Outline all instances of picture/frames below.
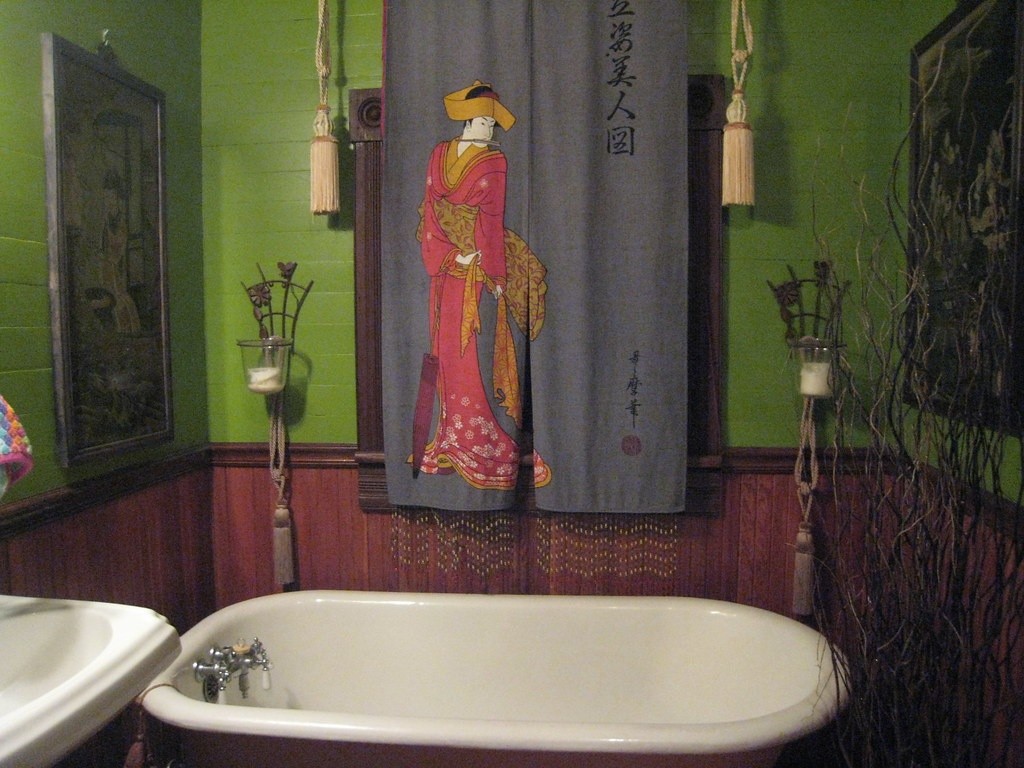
[42,30,175,467]
[904,0,1024,433]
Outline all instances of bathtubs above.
[0,591,182,767]
[141,588,856,768]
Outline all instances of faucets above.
[231,636,273,698]
